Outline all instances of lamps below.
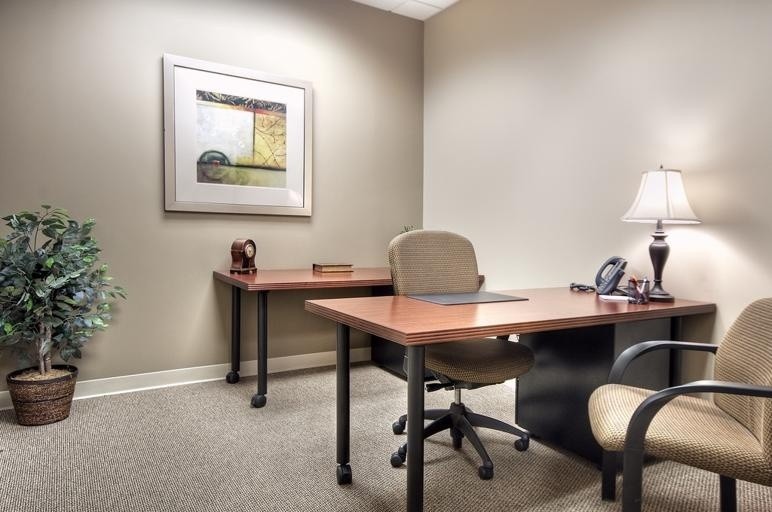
[621,164,702,302]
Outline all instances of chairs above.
[389,228,534,481]
[586,296,771,511]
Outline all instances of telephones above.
[596,256,628,295]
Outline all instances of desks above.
[304,287,716,512]
[214,267,487,408]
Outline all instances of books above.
[312,262,354,274]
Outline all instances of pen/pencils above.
[630,275,648,304]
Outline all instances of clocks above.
[230,239,257,275]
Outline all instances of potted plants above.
[0,202,127,426]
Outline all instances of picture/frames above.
[160,50,315,220]
